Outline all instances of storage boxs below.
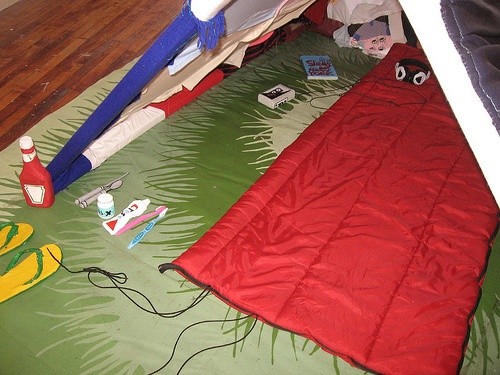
[257,85,296,110]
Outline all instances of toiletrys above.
[18,136,55,208]
[96,192,151,235]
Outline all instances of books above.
[299,54,341,83]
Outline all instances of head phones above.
[394,58,431,86]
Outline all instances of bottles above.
[18,136,55,209]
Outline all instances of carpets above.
[1,30,498,375]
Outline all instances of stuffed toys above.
[350,20,393,61]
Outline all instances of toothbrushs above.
[115,205,169,249]
